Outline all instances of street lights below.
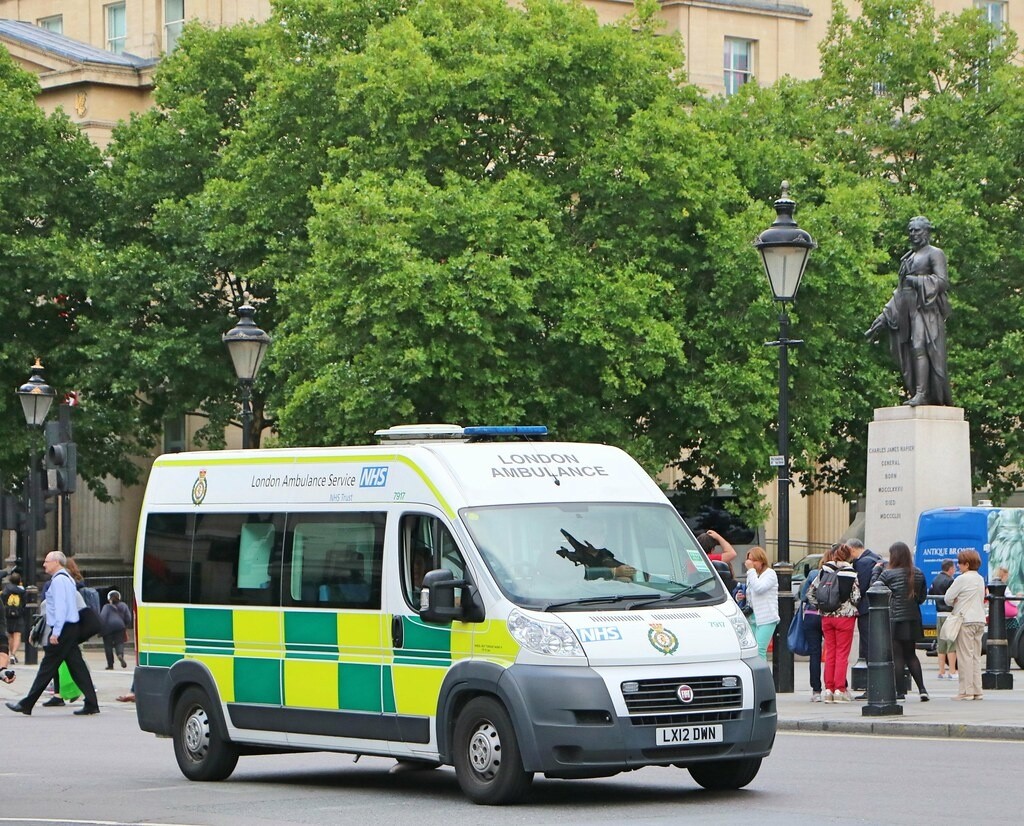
[224,290,270,449]
[752,179,819,692]
[14,357,58,665]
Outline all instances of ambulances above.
[130,424,779,808]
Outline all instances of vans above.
[912,505,1024,670]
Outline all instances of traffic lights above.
[49,443,67,490]
[40,489,56,528]
[15,511,24,534]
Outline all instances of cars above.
[788,553,830,612]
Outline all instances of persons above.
[985,567,1023,672]
[800,538,890,704]
[5,551,100,716]
[0,567,26,664]
[412,550,428,608]
[944,551,987,701]
[928,559,959,680]
[0,597,16,683]
[734,547,780,661]
[685,531,739,592]
[865,216,955,407]
[98,591,132,670]
[870,541,930,702]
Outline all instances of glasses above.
[747,557,758,563]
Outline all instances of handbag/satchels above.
[940,613,964,642]
[124,632,130,643]
[786,606,809,655]
[77,608,104,645]
[27,614,46,648]
[1004,600,1018,617]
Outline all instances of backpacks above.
[815,566,846,613]
[3,587,26,620]
[79,586,100,617]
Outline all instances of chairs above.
[318,583,369,603]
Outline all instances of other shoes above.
[115,694,136,702]
[810,690,868,703]
[6,703,32,715]
[43,682,79,707]
[93,684,98,692]
[950,693,984,701]
[118,655,127,668]
[896,693,905,701]
[938,671,959,679]
[8,654,16,664]
[73,701,100,715]
[105,666,113,670]
[919,689,929,702]
